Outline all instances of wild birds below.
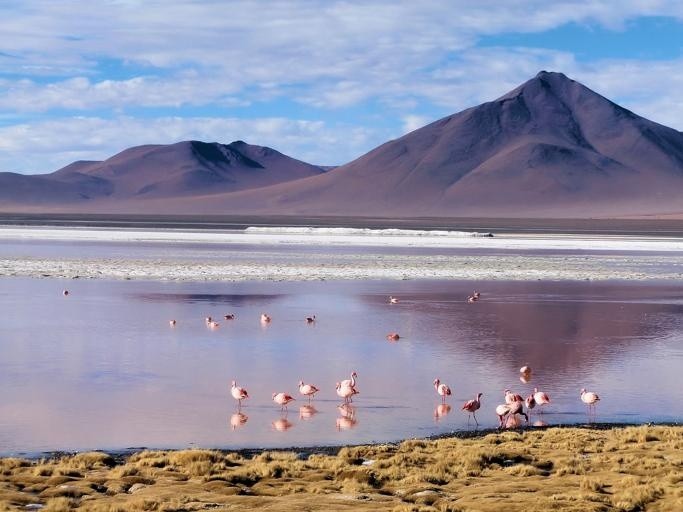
[385,333,399,342]
[467,289,480,303]
[305,315,316,324]
[580,388,601,416]
[299,400,319,422]
[224,315,234,321]
[336,401,357,430]
[229,407,247,434]
[334,372,359,400]
[230,380,248,406]
[387,295,399,306]
[206,317,220,330]
[169,320,176,326]
[272,392,295,412]
[271,411,291,434]
[519,365,531,374]
[519,374,531,384]
[433,378,451,402]
[433,402,451,423]
[297,380,319,400]
[260,314,271,327]
[464,393,483,425]
[497,387,551,429]
